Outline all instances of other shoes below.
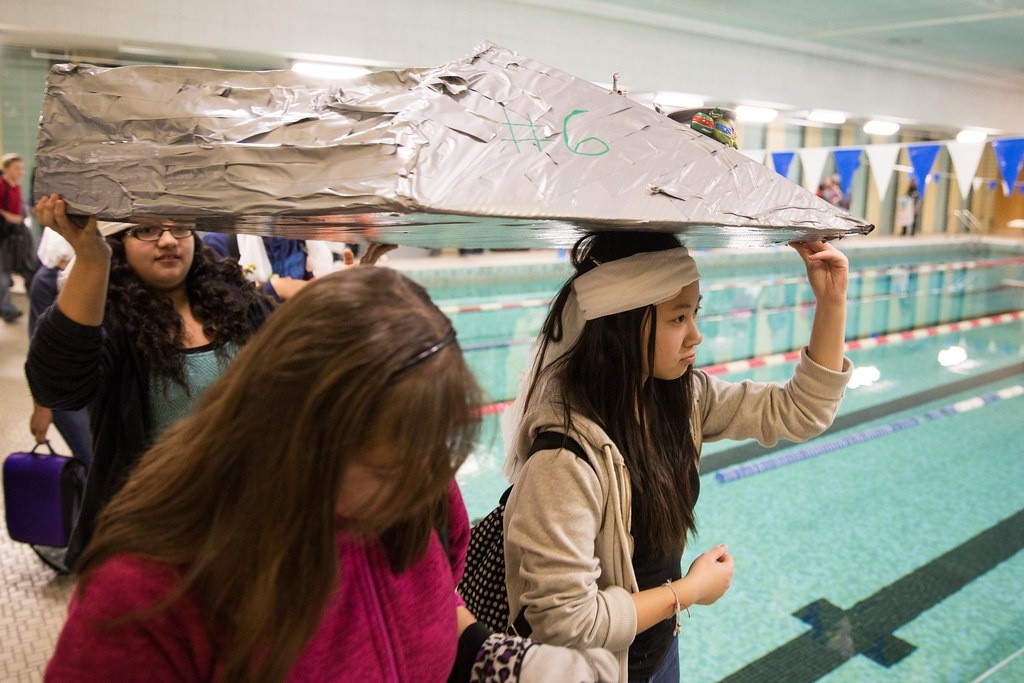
[0,309,24,321]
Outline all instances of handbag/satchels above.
[2,441,88,575]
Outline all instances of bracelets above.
[661,579,692,638]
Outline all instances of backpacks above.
[457,431,598,642]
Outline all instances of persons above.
[502,221,849,683]
[815,173,850,208]
[41,265,596,683]
[26,232,94,472]
[196,228,356,303]
[24,192,399,565]
[0,153,46,321]
[898,179,920,234]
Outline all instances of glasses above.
[123,224,197,242]
